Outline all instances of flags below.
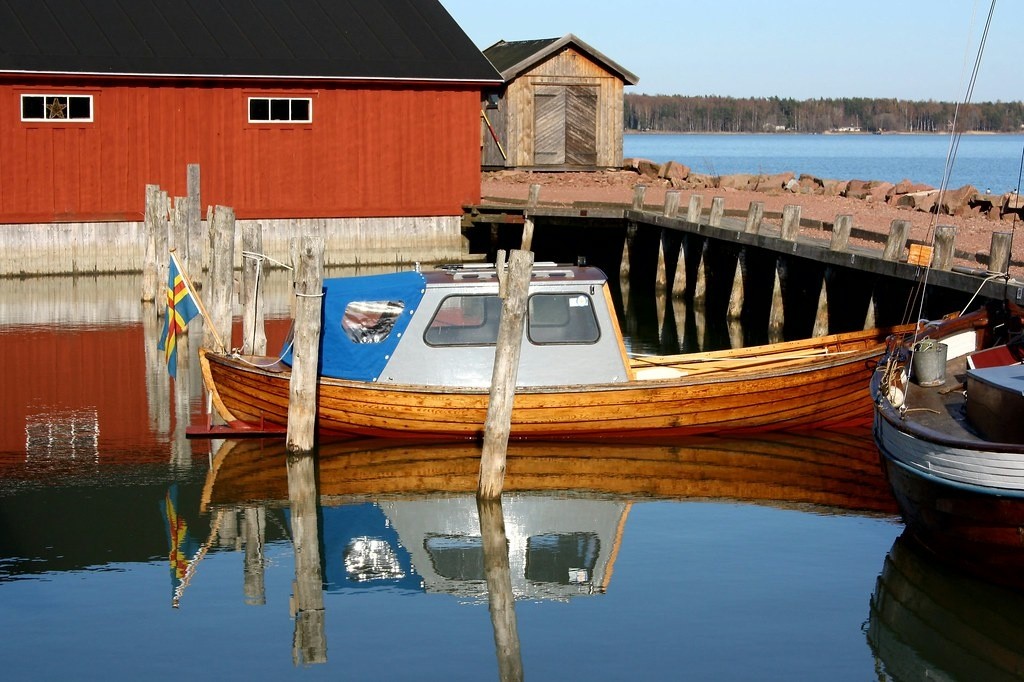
[158,256,200,382]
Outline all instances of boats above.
[870,0,1024,556]
[185,249,990,447]
[198,417,903,521]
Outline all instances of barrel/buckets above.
[908,343,948,387]
